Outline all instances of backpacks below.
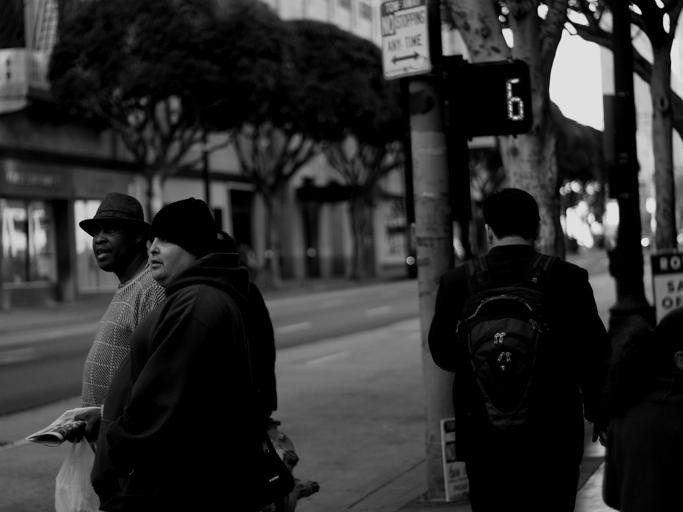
[454,249,562,434]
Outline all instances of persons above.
[425,187,609,512]
[72,198,280,512]
[49,191,164,512]
[602,306,682,512]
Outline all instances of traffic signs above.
[378,0,433,82]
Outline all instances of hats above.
[79,192,153,241]
[149,196,219,260]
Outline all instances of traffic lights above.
[464,57,534,139]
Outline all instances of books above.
[25,405,98,447]
